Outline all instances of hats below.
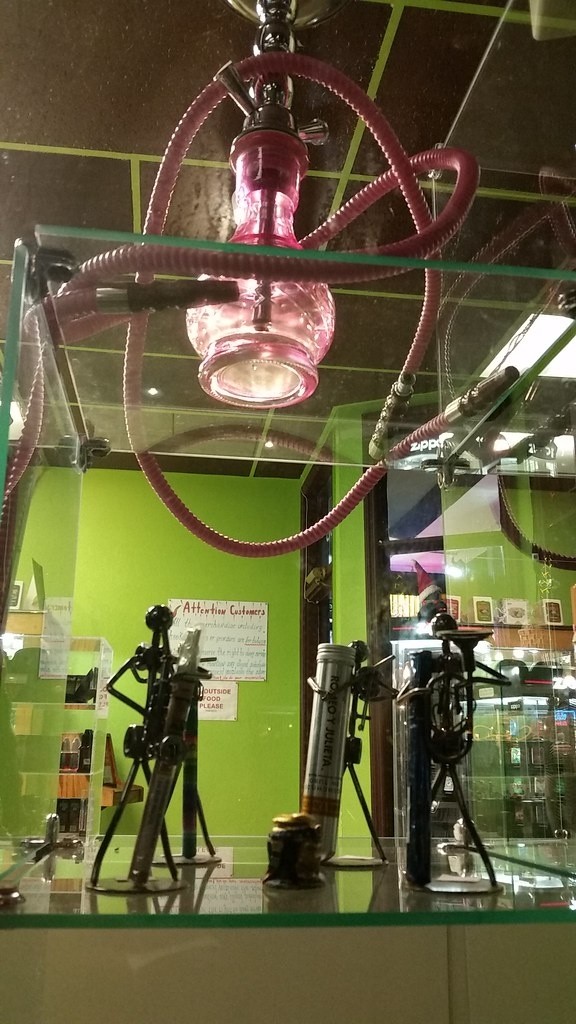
[409,559,441,604]
